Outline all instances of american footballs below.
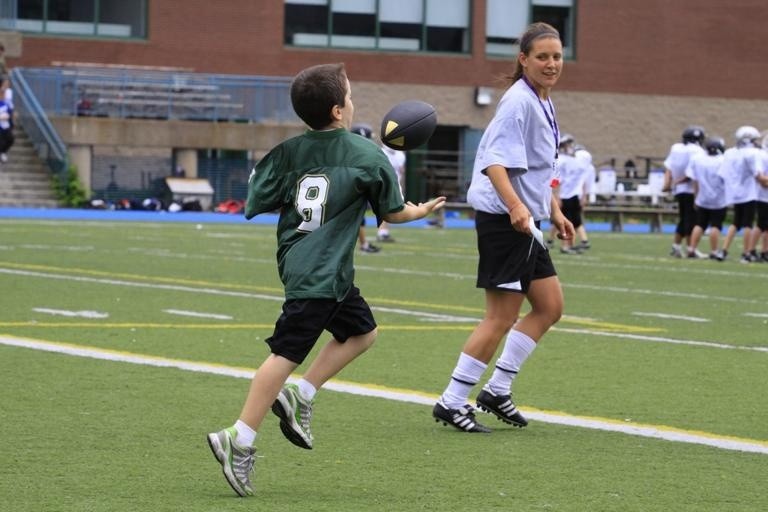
[380,101,436,150]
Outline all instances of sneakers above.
[429,396,491,434]
[267,383,315,451]
[668,240,767,266]
[205,426,258,499]
[475,382,528,428]
[376,235,395,244]
[358,241,382,255]
[559,238,593,257]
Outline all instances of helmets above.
[734,125,763,144]
[681,126,705,140]
[557,128,573,147]
[708,136,724,154]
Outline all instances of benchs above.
[51,52,247,126]
[410,143,735,233]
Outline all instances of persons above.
[207,63,447,497]
[662,126,768,264]
[433,21,577,434]
[0,46,15,162]
[349,125,406,252]
[551,133,596,254]
